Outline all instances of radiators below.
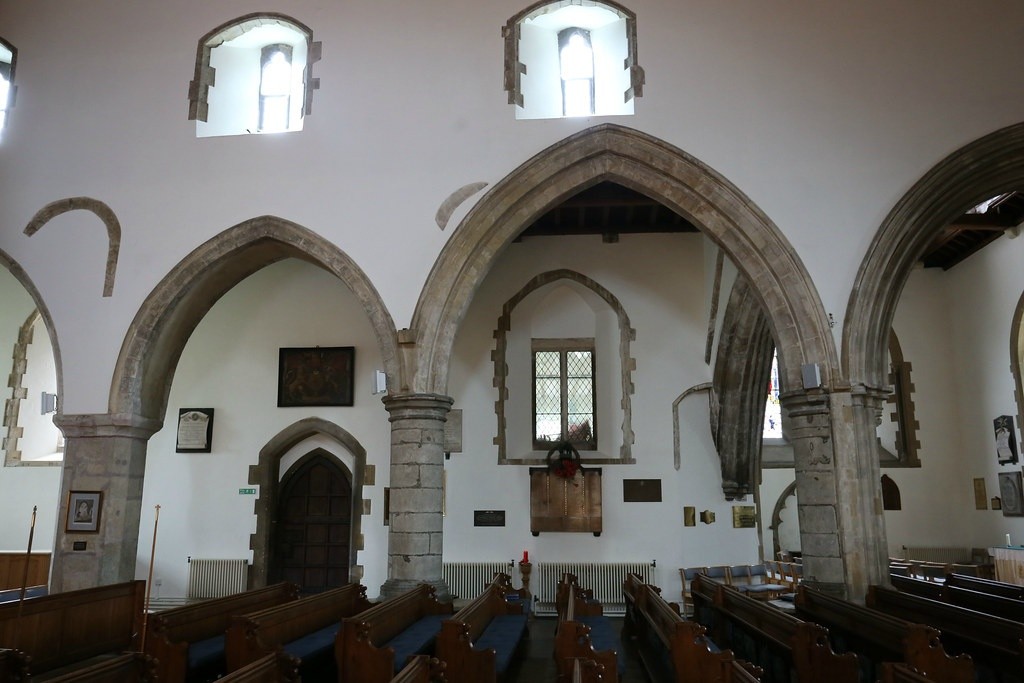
[902,544,972,565]
[537,561,653,603]
[188,556,249,599]
[442,560,512,599]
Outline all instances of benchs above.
[0,568,1024,683]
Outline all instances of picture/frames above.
[276,345,355,408]
[65,489,103,534]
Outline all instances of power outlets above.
[155,576,162,586]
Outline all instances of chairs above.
[678,547,981,621]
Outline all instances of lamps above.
[40,391,56,416]
[801,363,821,390]
[371,370,387,394]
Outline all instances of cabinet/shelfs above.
[529,466,603,538]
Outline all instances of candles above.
[1005,534,1010,545]
[523,550,528,562]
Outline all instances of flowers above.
[553,462,577,481]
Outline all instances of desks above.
[993,545,1024,587]
